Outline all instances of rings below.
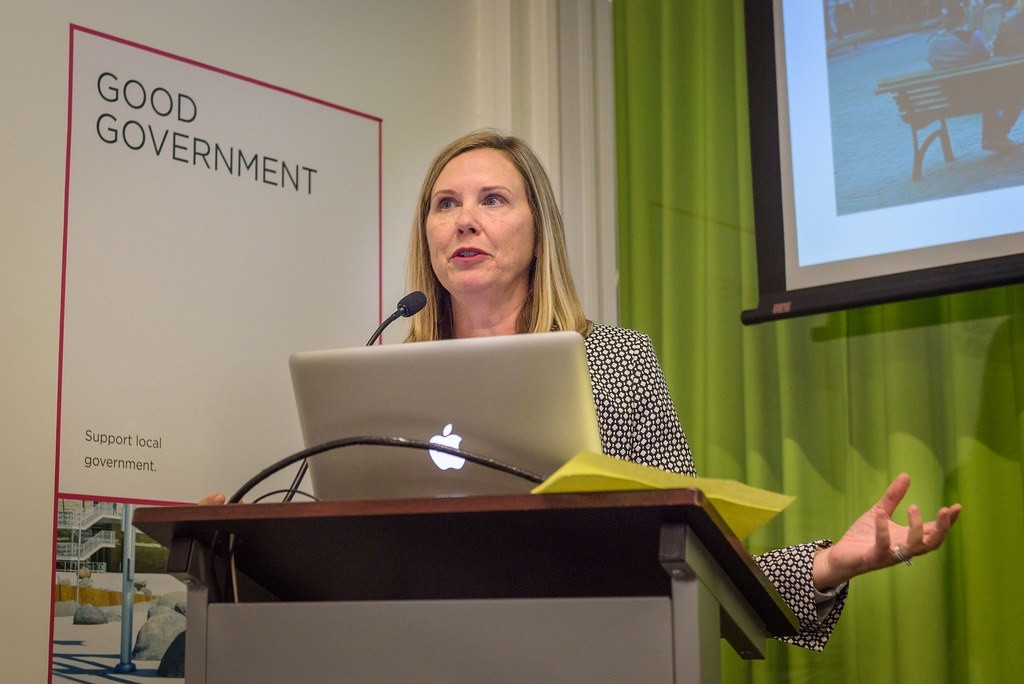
[894,546,912,566]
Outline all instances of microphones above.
[283,290,428,502]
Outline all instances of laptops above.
[289,330,603,504]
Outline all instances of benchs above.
[874,54,1023,179]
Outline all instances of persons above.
[198,128,962,654]
[925,0,1024,151]
